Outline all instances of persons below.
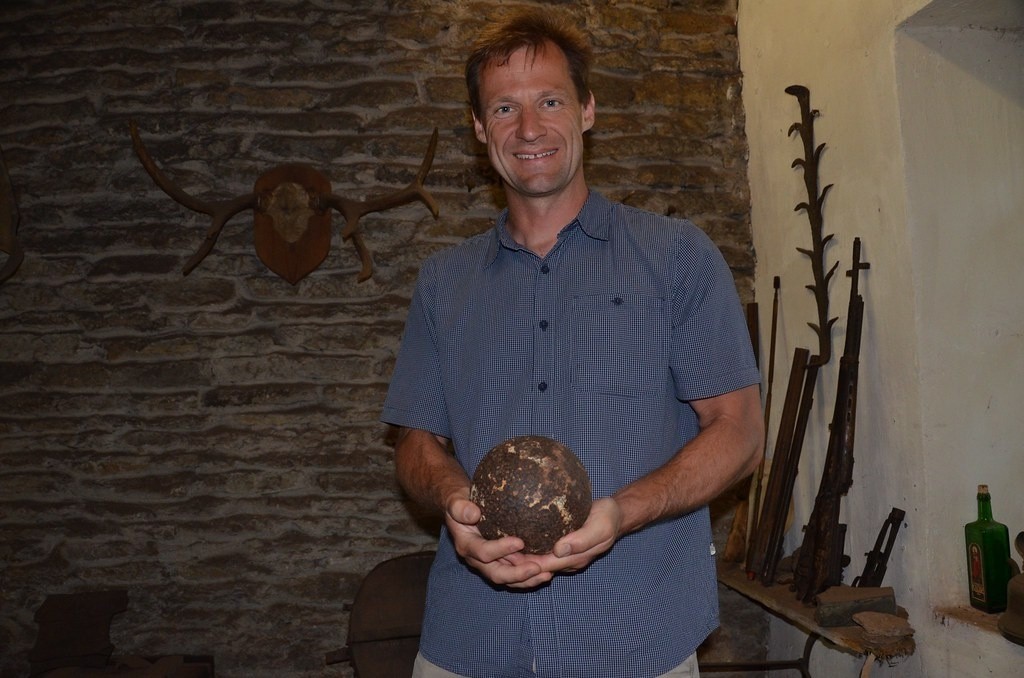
[378,13,766,678]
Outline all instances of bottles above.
[965,492,1011,614]
